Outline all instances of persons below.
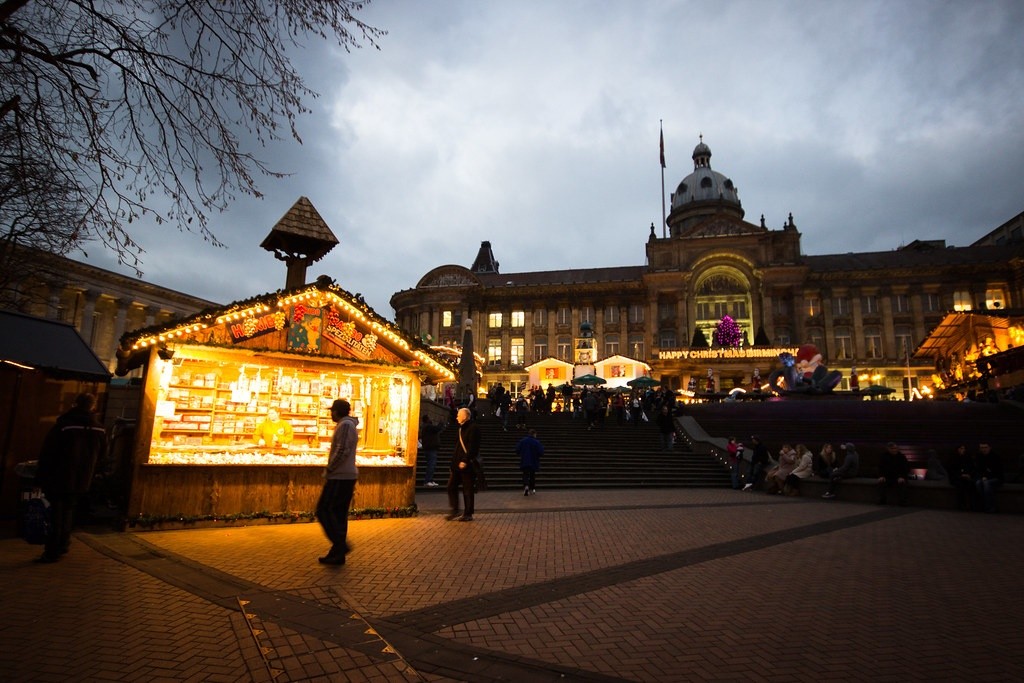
[422,415,444,486]
[656,406,672,449]
[883,441,914,507]
[253,406,294,447]
[767,443,813,496]
[985,383,1024,404]
[948,440,1004,514]
[445,407,481,521]
[812,443,858,501]
[445,382,685,432]
[515,429,544,496]
[316,398,358,565]
[90,419,136,515]
[32,392,108,563]
[726,435,769,491]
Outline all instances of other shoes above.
[455,514,472,523]
[532,489,536,496]
[823,492,835,499]
[446,512,460,520]
[524,486,530,497]
[317,544,352,566]
[33,550,66,562]
[789,489,800,497]
[423,481,439,487]
[743,483,752,491]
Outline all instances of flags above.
[659,131,666,168]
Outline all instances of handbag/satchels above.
[625,410,631,421]
[470,458,483,472]
[496,406,503,418]
[672,433,677,445]
[642,413,648,422]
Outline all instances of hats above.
[328,400,351,416]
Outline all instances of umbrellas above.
[861,385,896,400]
[626,376,664,391]
[571,374,607,388]
